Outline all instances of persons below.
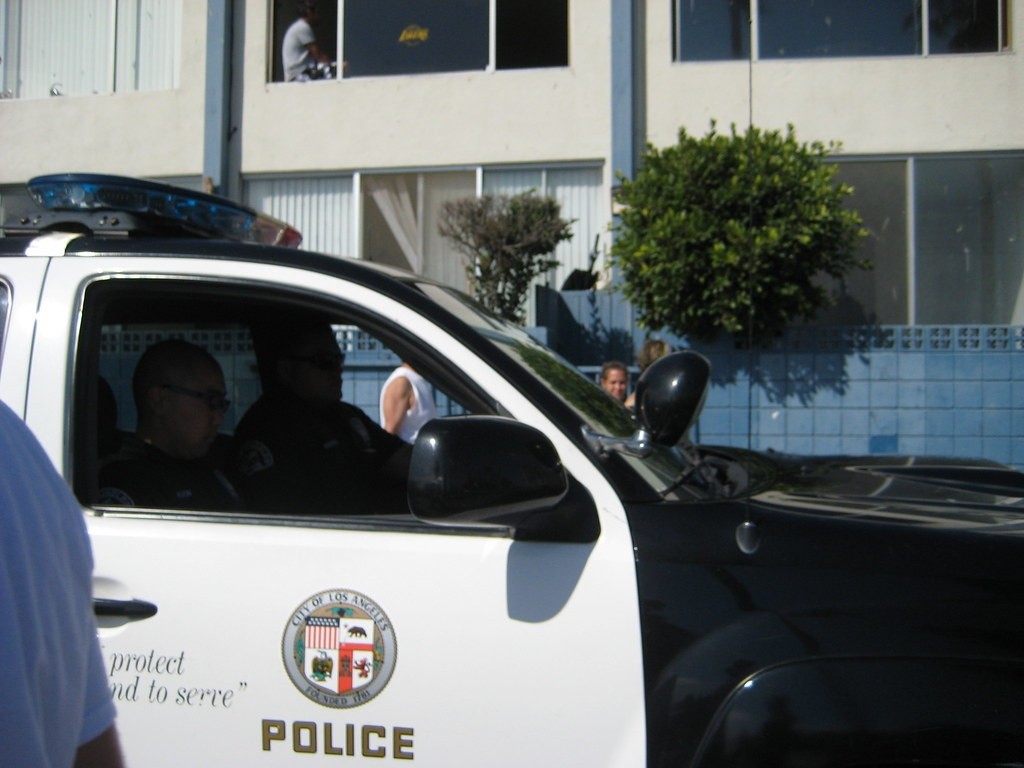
[234,308,413,515]
[281,0,350,82]
[106,339,234,513]
[599,363,627,401]
[625,340,670,408]
[379,361,439,445]
[0,401,127,768]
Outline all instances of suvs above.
[0,172,1023,768]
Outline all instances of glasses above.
[281,352,346,370]
[162,381,230,415]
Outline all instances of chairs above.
[74,366,137,508]
